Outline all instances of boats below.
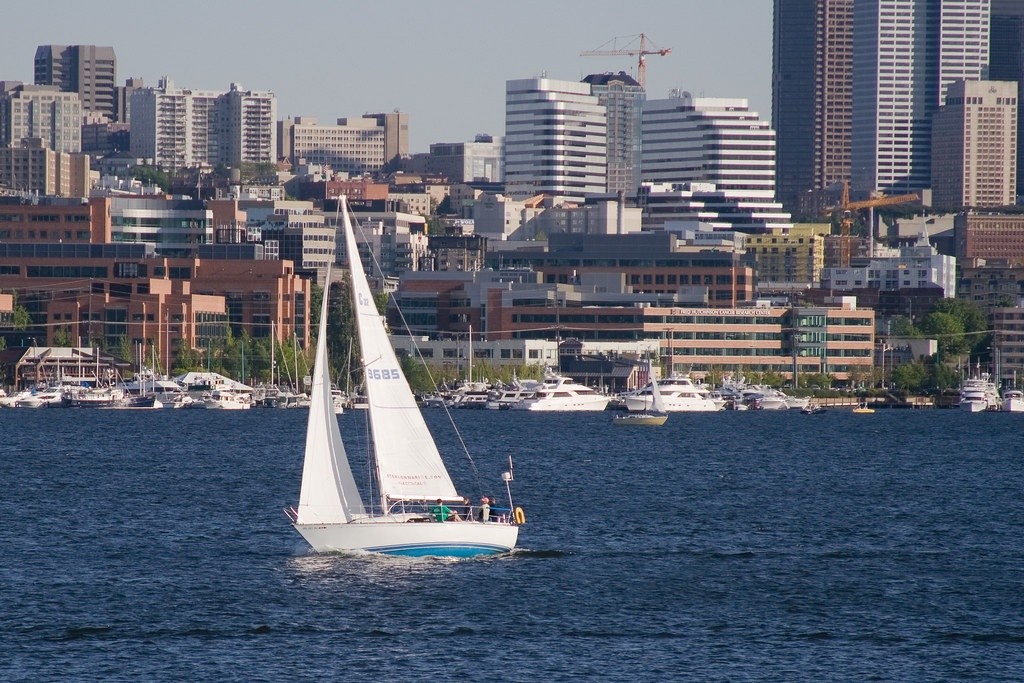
[852,401,876,415]
[1001,388,1024,413]
[957,376,1001,414]
[421,308,828,415]
[611,412,669,427]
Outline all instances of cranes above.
[577,32,673,88]
[820,177,922,269]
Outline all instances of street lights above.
[958,350,971,389]
[882,346,894,388]
[986,346,999,388]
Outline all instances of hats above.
[481,497,489,503]
[489,496,495,505]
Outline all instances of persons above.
[464,498,473,521]
[489,497,504,522]
[479,498,490,521]
[432,499,457,522]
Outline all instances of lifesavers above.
[515,507,525,524]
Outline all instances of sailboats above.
[281,194,523,560]
[0,316,370,416]
